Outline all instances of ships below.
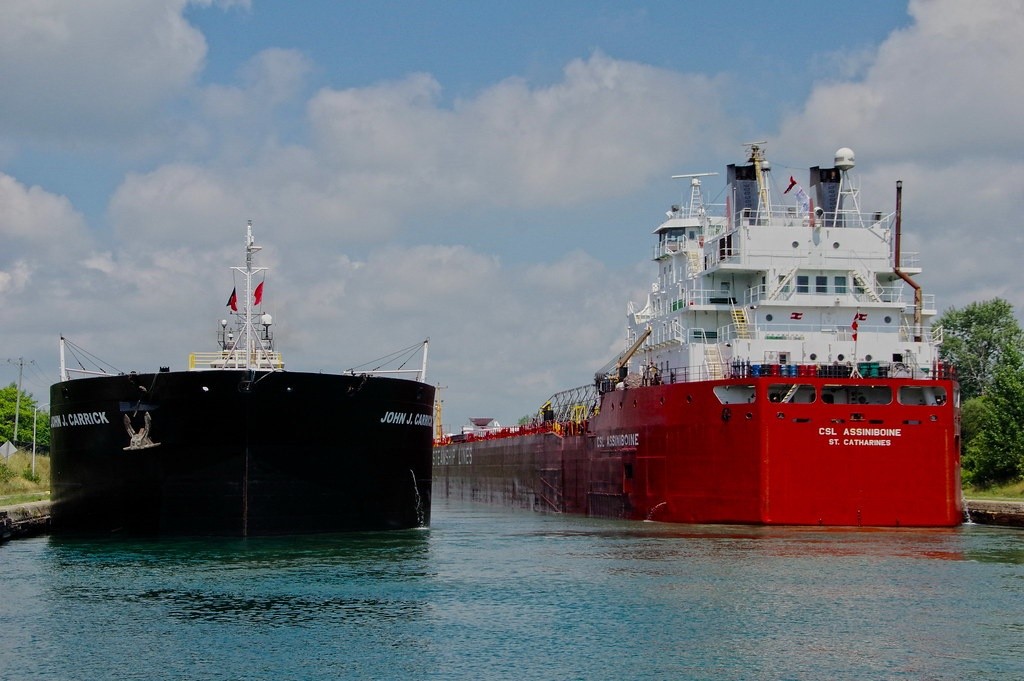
[50,217,436,547]
[433,135,966,526]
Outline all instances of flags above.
[783,176,797,195]
[253,281,263,306]
[853,313,858,321]
[852,333,857,342]
[794,188,807,204]
[851,321,857,331]
[858,314,867,321]
[226,289,237,312]
[790,313,802,320]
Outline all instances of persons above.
[564,425,584,437]
[653,372,675,386]
[225,338,237,351]
